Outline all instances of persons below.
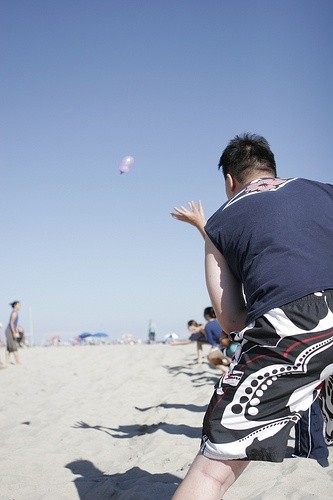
[4,301,22,368]
[204,306,226,352]
[187,319,209,361]
[13,326,24,347]
[207,330,241,375]
[171,133,333,500]
[148,319,155,343]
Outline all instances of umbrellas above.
[93,332,108,336]
[80,333,92,338]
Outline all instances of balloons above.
[119,156,134,174]
